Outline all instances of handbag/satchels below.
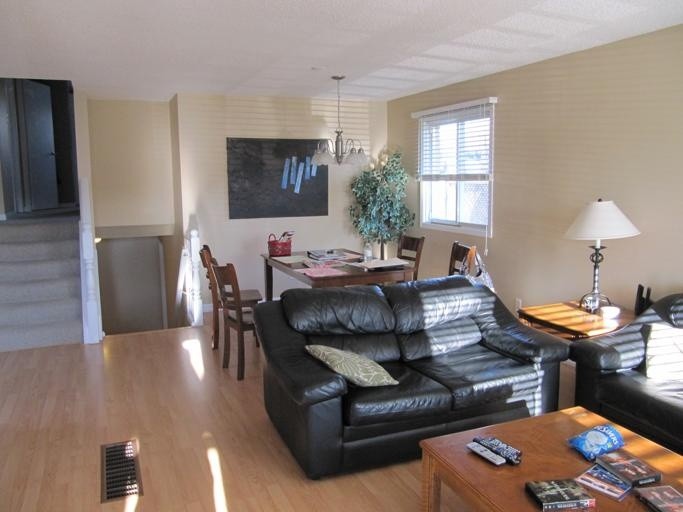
[459,249,494,293]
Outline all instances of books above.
[306,248,348,263]
[523,448,682,512]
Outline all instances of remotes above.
[473,435,520,464]
[486,436,522,456]
[467,442,506,465]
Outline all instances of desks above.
[260,248,416,302]
[517,300,635,341]
[419,406,683,512]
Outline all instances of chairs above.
[211,258,259,380]
[448,240,477,275]
[397,232,425,283]
[199,244,263,349]
[569,293,683,455]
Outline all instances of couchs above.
[252,275,570,480]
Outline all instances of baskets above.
[268,232,291,257]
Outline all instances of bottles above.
[363,242,373,271]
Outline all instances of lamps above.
[561,199,641,313]
[310,75,368,166]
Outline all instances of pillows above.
[280,274,483,387]
[646,322,683,380]
